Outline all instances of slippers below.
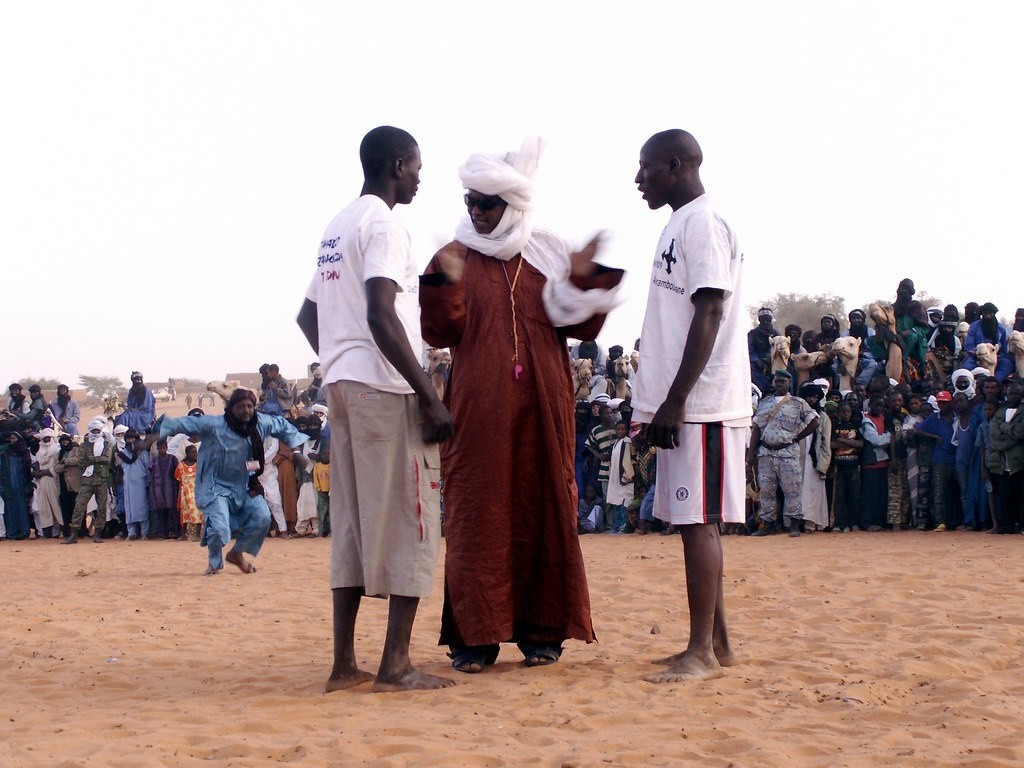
[205,562,223,576]
[225,552,256,573]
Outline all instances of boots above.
[93,529,103,543]
[61,528,78,543]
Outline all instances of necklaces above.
[502,255,523,380]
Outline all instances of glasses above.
[464,194,502,211]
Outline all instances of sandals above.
[526,646,560,666]
[446,647,486,672]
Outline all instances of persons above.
[418,134,626,674]
[630,129,754,683]
[296,125,457,693]
[0,278,1024,576]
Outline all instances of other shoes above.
[607,520,1018,537]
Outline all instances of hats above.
[1002,373,1021,383]
[776,369,792,378]
[936,391,951,402]
[630,420,641,426]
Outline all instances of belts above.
[760,441,794,451]
